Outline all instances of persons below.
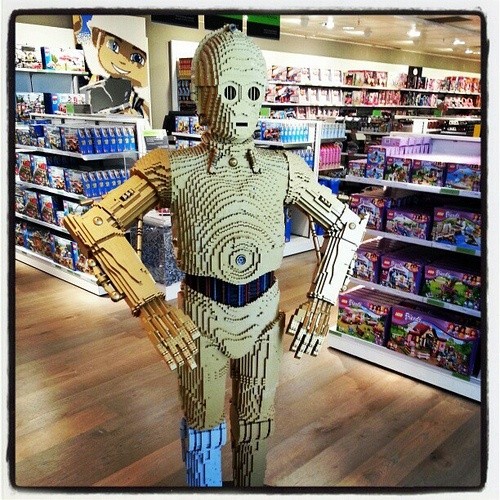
[61,24,369,486]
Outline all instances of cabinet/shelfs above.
[166,110,348,259]
[327,130,481,403]
[169,41,481,156]
[16,68,153,296]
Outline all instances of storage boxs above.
[16,44,481,381]
